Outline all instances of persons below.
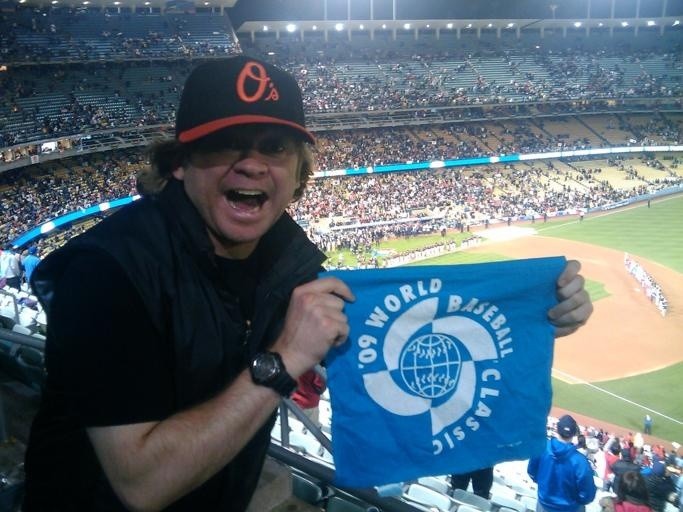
[448,399,683,511]
[17,50,593,511]
[285,354,325,433]
[1,0,683,292]
[647,289,671,319]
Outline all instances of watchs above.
[245,336,297,399]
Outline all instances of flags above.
[315,261,563,485]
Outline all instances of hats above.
[557,416,577,437]
[176,56,316,143]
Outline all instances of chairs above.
[269,415,537,511]
[0,283,47,399]
